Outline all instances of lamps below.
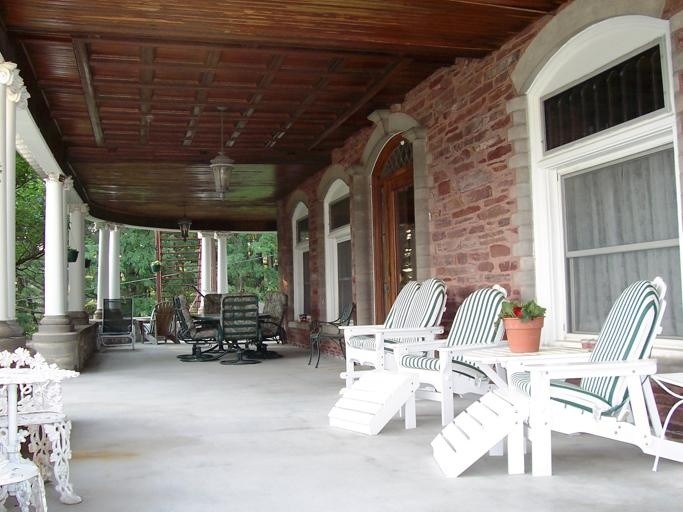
[178,204,192,242]
[209,106,235,201]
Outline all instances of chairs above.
[0,347,81,512]
[308,303,355,368]
[96,291,288,365]
[429,277,672,478]
[329,277,516,439]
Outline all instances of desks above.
[462,344,596,454]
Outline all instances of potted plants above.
[497,295,550,356]
